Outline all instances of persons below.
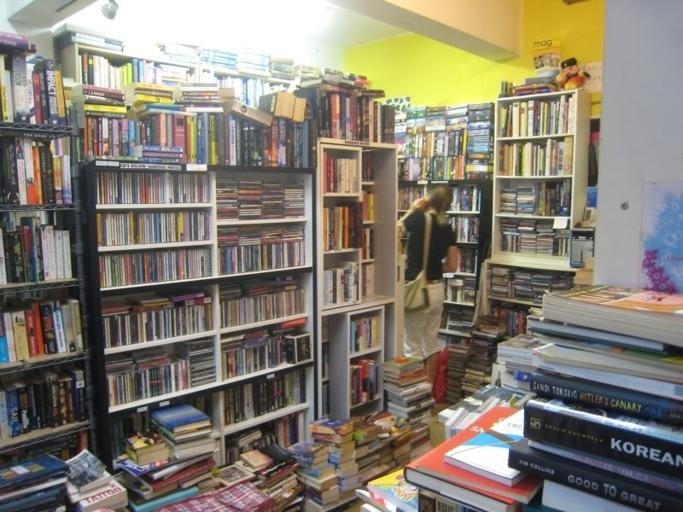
[395,187,458,361]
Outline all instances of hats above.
[560,57,578,68]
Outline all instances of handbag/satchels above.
[403,209,431,314]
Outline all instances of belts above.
[426,279,450,284]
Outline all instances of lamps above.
[102,0,119,19]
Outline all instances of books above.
[322,259,359,309]
[316,85,395,146]
[348,316,383,353]
[217,176,306,220]
[486,266,573,305]
[218,327,313,379]
[572,232,595,270]
[112,395,216,460]
[322,317,334,422]
[78,52,314,167]
[154,32,370,90]
[362,226,375,260]
[0,365,87,441]
[99,247,211,289]
[104,337,218,409]
[397,181,479,213]
[499,69,557,99]
[218,224,308,274]
[364,263,376,301]
[212,357,435,512]
[95,170,210,204]
[220,274,308,328]
[323,199,361,252]
[322,148,359,193]
[497,178,572,217]
[112,404,215,512]
[94,210,211,246]
[399,212,480,243]
[495,216,569,256]
[395,102,494,180]
[0,210,77,285]
[220,366,311,427]
[362,149,377,183]
[363,189,376,224]
[347,284,682,512]
[1,291,83,365]
[0,448,129,512]
[0,29,78,131]
[1,132,75,208]
[498,94,573,139]
[224,410,308,468]
[454,245,477,275]
[100,284,214,350]
[0,430,90,467]
[497,137,573,177]
[445,276,475,303]
[51,24,125,52]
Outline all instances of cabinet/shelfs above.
[491,88,591,263]
[310,135,401,420]
[483,257,594,340]
[398,179,493,345]
[81,159,315,476]
[1,121,103,467]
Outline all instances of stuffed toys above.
[557,57,590,92]
